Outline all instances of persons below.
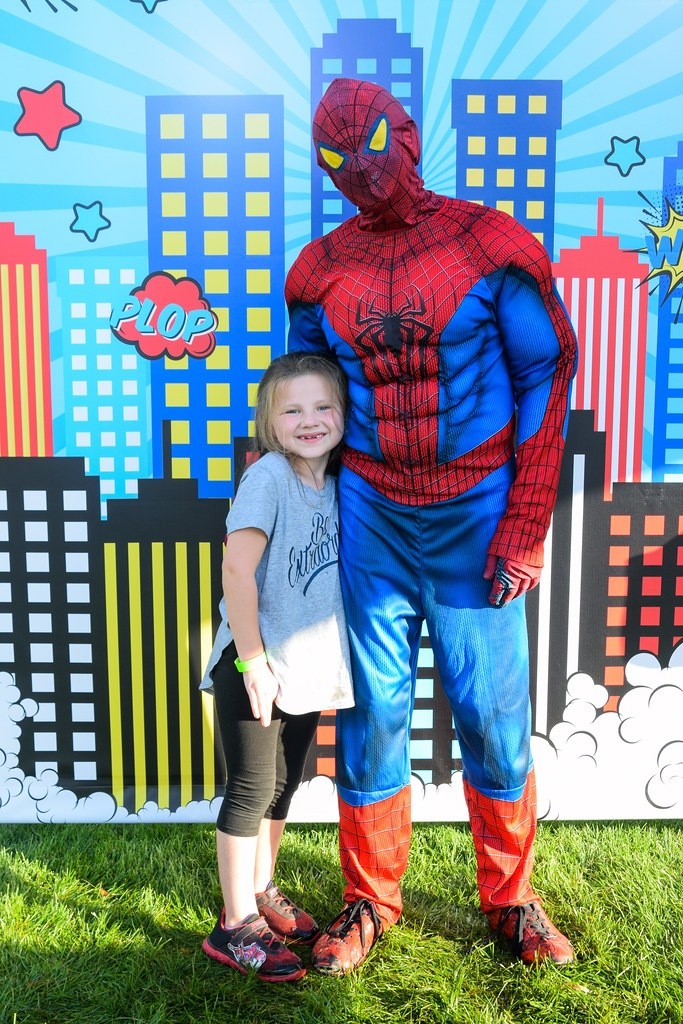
[199,356,356,983]
[286,76,577,973]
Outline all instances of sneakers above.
[310,898,382,976]
[254,881,319,946]
[201,904,306,982]
[488,899,574,965]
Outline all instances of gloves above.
[483,557,537,607]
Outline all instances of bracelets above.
[234,651,267,672]
